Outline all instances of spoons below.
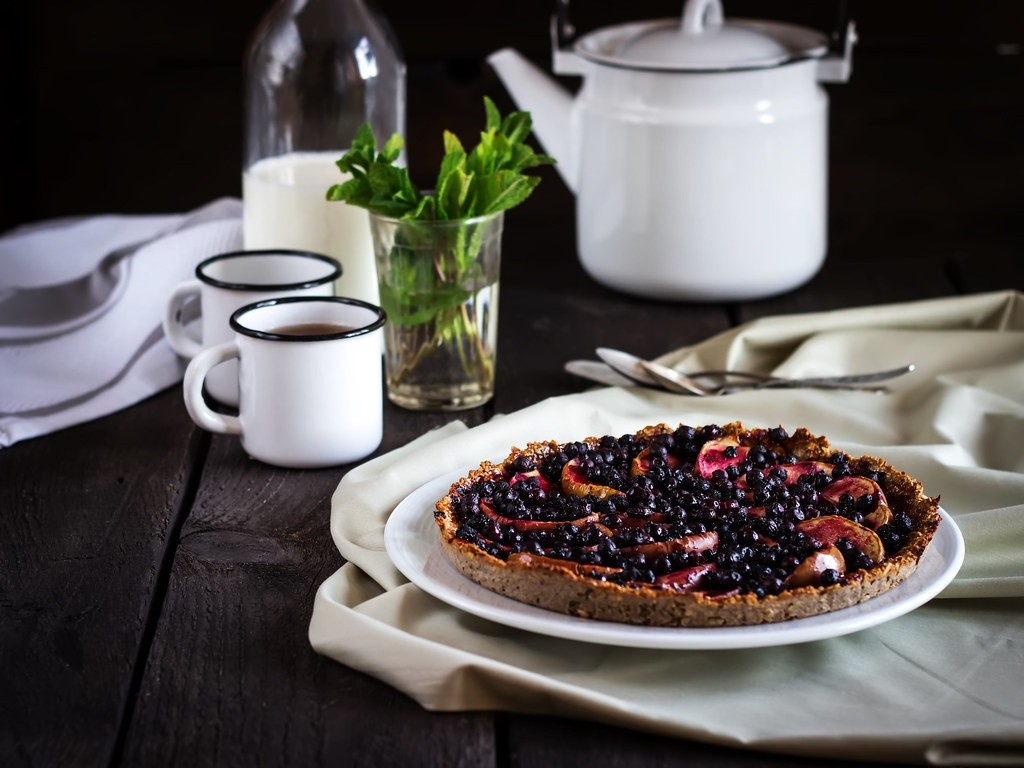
[564,346,916,396]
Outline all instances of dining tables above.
[0,198,820,768]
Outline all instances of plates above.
[384,460,966,649]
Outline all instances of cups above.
[163,249,342,404]
[370,190,504,411]
[183,296,384,467]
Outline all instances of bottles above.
[241,0,405,309]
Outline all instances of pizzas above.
[435,425,942,627]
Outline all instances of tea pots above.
[486,0,859,301]
